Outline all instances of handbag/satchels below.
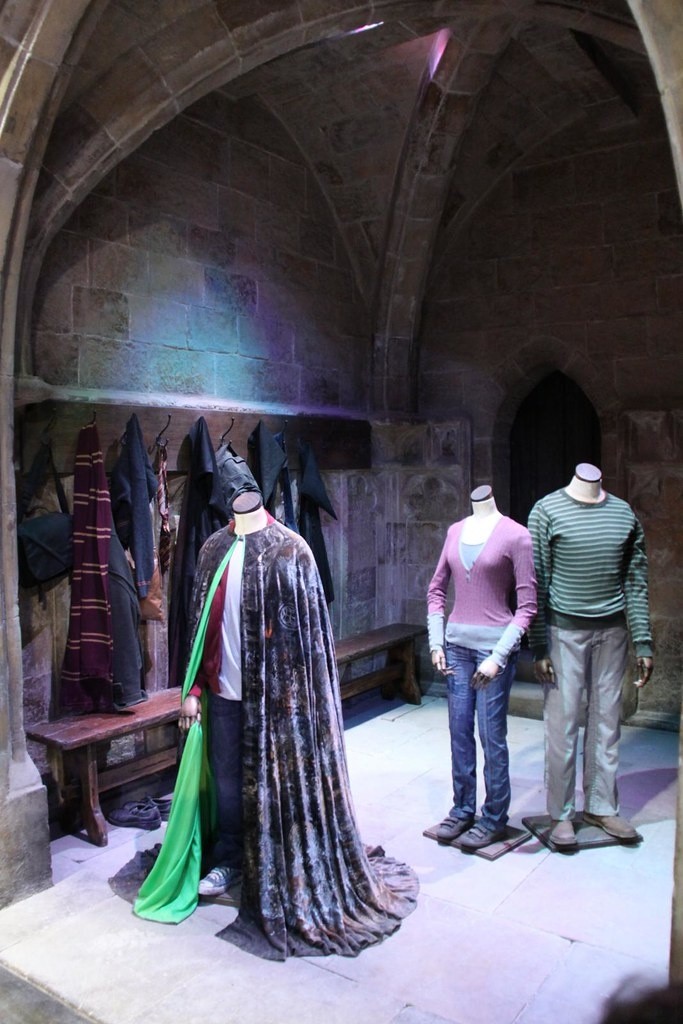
[18,511,74,590]
[121,548,164,624]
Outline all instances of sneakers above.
[199,864,242,895]
[436,816,474,839]
[460,823,507,849]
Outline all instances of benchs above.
[335,623,427,705]
[25,686,183,847]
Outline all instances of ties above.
[157,443,171,588]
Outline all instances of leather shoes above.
[582,810,638,840]
[107,795,172,830]
[548,820,578,847]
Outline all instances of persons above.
[528,464,655,845]
[116,491,420,961]
[426,484,538,847]
